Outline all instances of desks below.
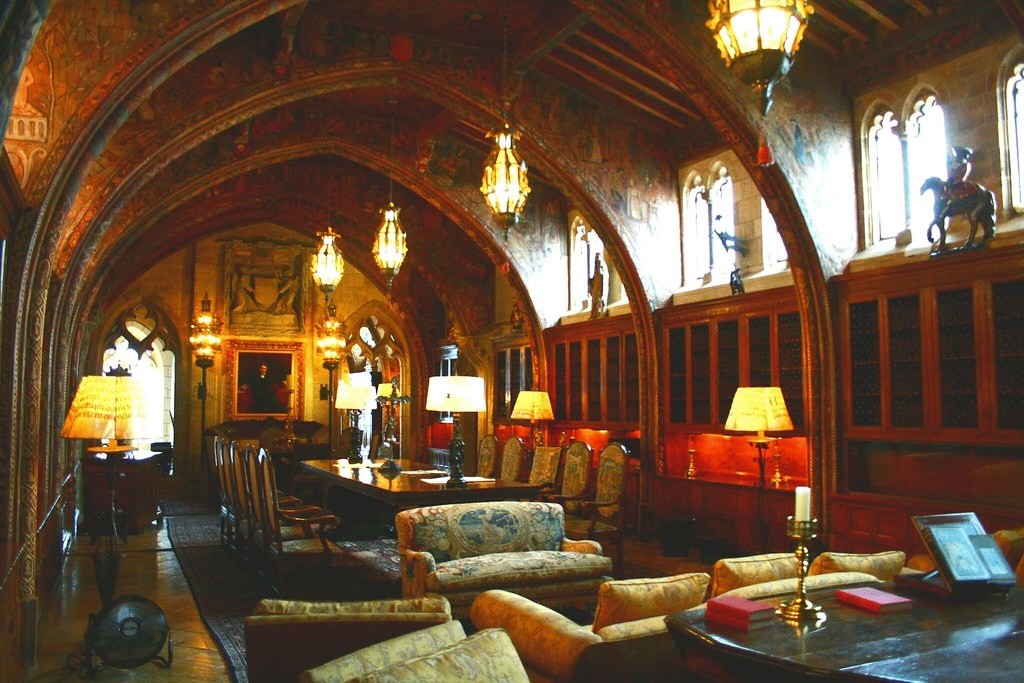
[300,455,544,513]
[664,569,1021,682]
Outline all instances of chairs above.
[476,435,631,579]
[339,428,364,459]
[204,430,355,600]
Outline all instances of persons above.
[274,265,295,314]
[231,265,255,312]
[940,147,972,218]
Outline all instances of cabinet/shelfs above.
[653,286,806,554]
[81,449,164,535]
[491,333,533,480]
[543,313,642,531]
[826,243,1024,567]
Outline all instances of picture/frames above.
[222,238,309,338]
[224,339,306,422]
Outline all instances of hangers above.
[159,500,236,515]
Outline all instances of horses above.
[920,176,998,250]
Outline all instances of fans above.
[85,595,173,681]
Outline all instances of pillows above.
[593,573,711,633]
[713,550,797,599]
[806,550,905,581]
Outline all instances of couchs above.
[240,501,1024,683]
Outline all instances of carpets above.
[167,516,718,683]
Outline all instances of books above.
[704,595,775,631]
[834,587,912,613]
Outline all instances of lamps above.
[59,375,163,606]
[480,16,530,276]
[509,391,554,448]
[308,157,344,323]
[335,378,369,463]
[183,290,223,483]
[426,376,486,489]
[723,386,795,554]
[315,299,348,459]
[706,1,814,167]
[372,98,410,310]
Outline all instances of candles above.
[794,487,812,521]
